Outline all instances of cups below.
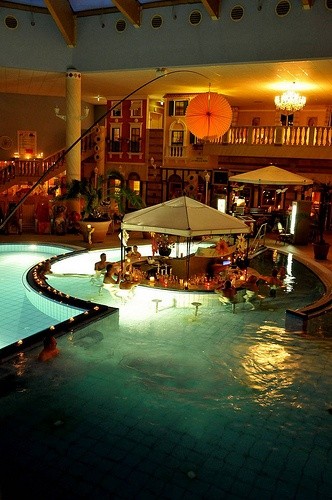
[150,277,154,286]
[179,278,187,289]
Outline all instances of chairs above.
[275,222,293,246]
[34,200,54,234]
[4,200,23,234]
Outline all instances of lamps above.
[273,81,308,114]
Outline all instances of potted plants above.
[53,168,149,243]
[308,180,332,259]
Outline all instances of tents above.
[119,196,251,286]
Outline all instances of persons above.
[110,274,139,302]
[38,336,61,363]
[98,264,123,294]
[240,275,259,311]
[38,260,52,280]
[86,227,95,251]
[125,244,141,262]
[95,253,118,271]
[259,265,289,297]
[214,281,237,305]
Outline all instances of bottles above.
[156,265,178,287]
